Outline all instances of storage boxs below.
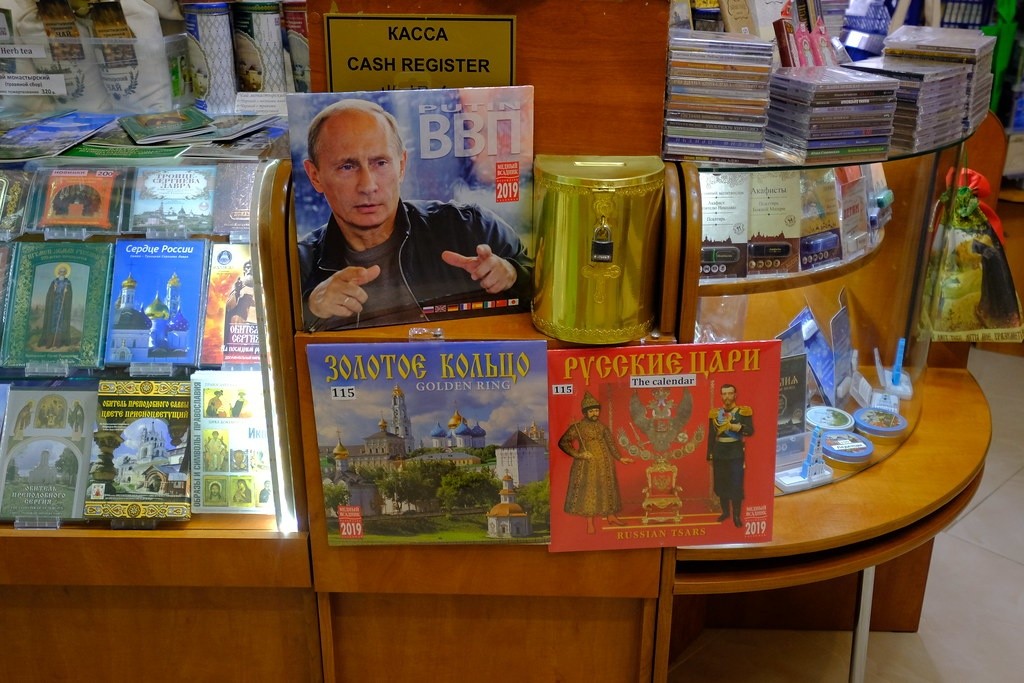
[535,153,666,346]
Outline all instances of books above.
[0,107,289,523]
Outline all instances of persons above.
[297,99,534,332]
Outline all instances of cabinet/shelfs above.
[973,187,1024,358]
[0,159,325,683]
[651,127,994,683]
[288,153,683,683]
